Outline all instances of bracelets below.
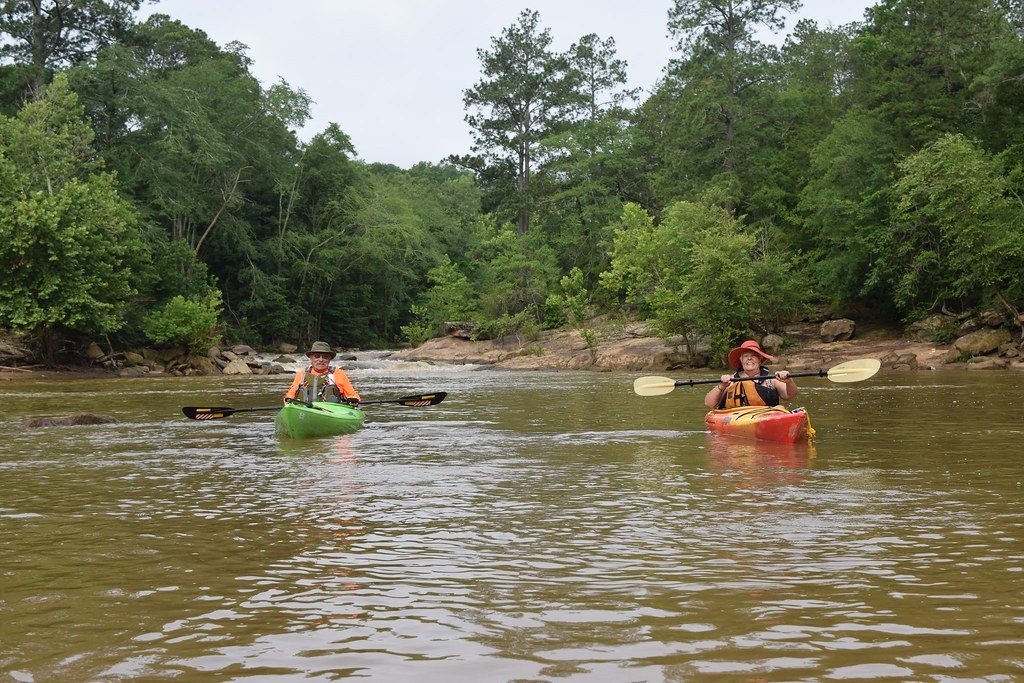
[718,385,724,391]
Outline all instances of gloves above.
[347,398,358,407]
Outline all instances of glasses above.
[314,354,328,359]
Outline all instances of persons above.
[705,340,798,411]
[283,341,361,408]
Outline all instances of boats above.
[703,406,807,444]
[274,399,365,438]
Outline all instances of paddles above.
[181,387,448,424]
[633,357,884,398]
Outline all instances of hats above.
[728,340,773,370]
[306,341,336,360]
